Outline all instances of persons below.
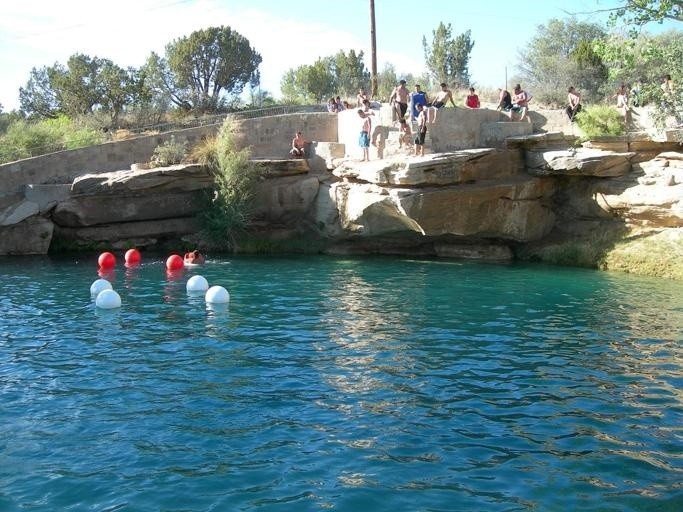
[564,86,582,125]
[184,249,205,264]
[289,132,313,159]
[327,80,532,163]
[611,72,675,122]
